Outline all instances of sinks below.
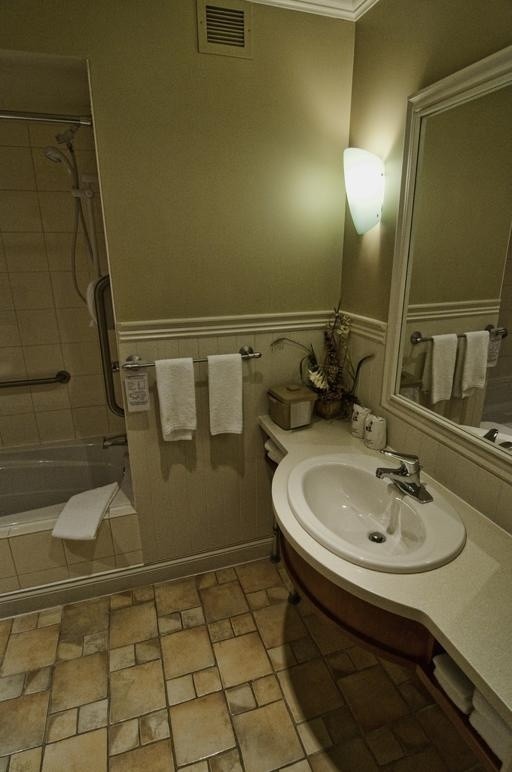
[1,446,133,536]
[462,424,511,451]
[288,454,466,573]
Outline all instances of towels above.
[50,482,120,542]
[154,356,198,444]
[421,329,491,406]
[431,654,508,763]
[206,351,244,437]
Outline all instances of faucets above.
[102,434,126,451]
[376,449,433,504]
[484,428,512,448]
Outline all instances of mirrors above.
[379,43,512,486]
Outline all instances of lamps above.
[341,146,384,236]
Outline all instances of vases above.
[315,399,343,420]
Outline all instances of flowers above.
[269,298,375,401]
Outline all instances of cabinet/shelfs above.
[275,525,511,772]
